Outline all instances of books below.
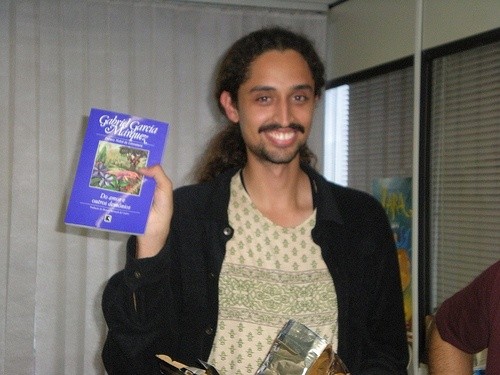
[61,104,170,239]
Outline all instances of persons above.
[423,254,500,375]
[95,24,412,375]
